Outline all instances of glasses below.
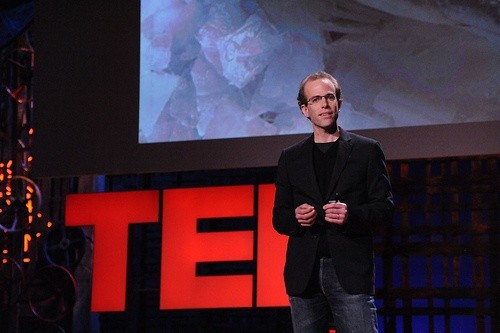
[307,94,336,105]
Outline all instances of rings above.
[336,214,339,219]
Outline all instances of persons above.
[272,72,395,333]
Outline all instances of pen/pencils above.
[335,193,339,202]
